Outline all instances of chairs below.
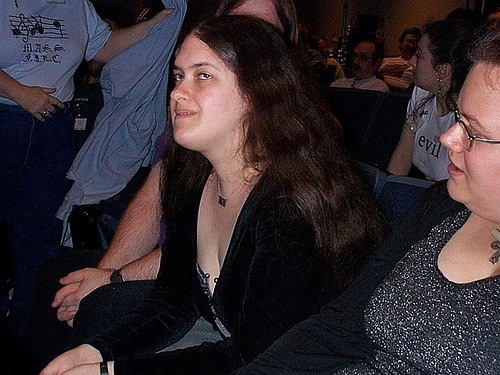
[321,84,437,310]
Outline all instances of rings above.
[42,109,47,116]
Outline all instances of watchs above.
[109,268,125,285]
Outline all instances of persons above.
[374,25,425,89]
[35,12,393,375]
[57,16,119,193]
[309,31,345,86]
[346,10,386,77]
[385,19,482,189]
[330,36,390,93]
[0,0,173,331]
[232,16,500,375]
[19,0,308,375]
[440,7,485,90]
[476,3,500,82]
[292,21,326,88]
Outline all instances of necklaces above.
[213,159,263,209]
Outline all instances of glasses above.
[452,100,500,148]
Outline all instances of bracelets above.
[100,360,110,375]
